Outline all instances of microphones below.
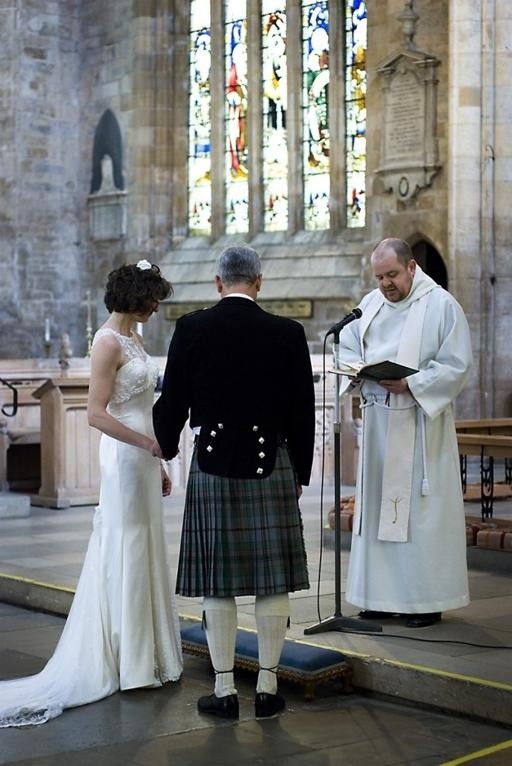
[330,307,362,333]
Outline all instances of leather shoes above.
[358,611,442,628]
[255,693,285,720]
[197,693,239,718]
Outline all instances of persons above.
[331,238,475,627]
[150,247,316,721]
[1,260,185,728]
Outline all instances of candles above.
[86,290,92,325]
[137,322,143,337]
[45,318,50,341]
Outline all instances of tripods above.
[304,332,383,635]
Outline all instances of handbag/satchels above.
[197,414,277,479]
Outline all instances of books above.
[327,358,418,386]
